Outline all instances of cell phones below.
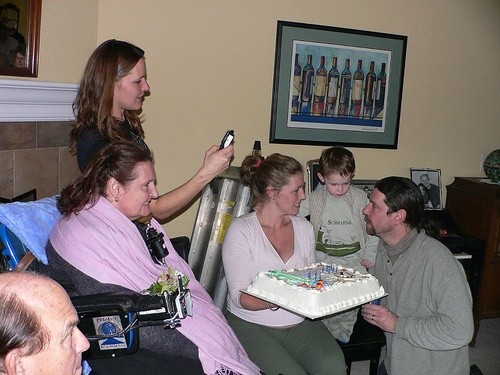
[219,130,234,150]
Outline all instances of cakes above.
[248,262,385,317]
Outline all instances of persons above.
[361,177,474,375]
[70,39,234,221]
[222,153,346,375]
[45,141,262,375]
[0,271,90,375]
[417,174,440,209]
[15,45,26,67]
[299,146,379,347]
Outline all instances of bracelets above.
[266,302,280,311]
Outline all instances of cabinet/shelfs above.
[447,174,500,320]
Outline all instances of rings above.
[372,315,374,320]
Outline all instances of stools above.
[335,311,386,375]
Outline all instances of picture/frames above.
[350,180,379,198]
[306,159,319,192]
[269,20,409,150]
[409,168,443,211]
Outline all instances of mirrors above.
[0,0,42,78]
